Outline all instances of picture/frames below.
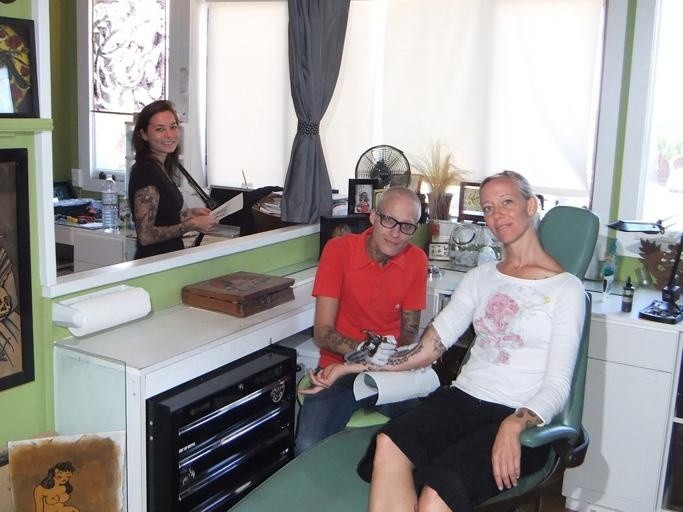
[348,179,378,215]
[456,182,485,221]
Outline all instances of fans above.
[355,145,411,188]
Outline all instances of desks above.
[421,268,683,512]
[51,251,322,512]
[54,219,240,273]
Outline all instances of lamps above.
[605,210,683,326]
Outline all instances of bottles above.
[602,265,614,303]
[622,277,634,312]
[102,171,118,233]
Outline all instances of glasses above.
[375,209,417,236]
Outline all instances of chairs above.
[227,206,600,512]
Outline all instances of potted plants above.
[412,139,470,220]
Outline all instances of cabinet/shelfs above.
[0,113,58,467]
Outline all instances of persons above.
[295,186,428,456]
[128,101,218,259]
[299,170,585,508]
